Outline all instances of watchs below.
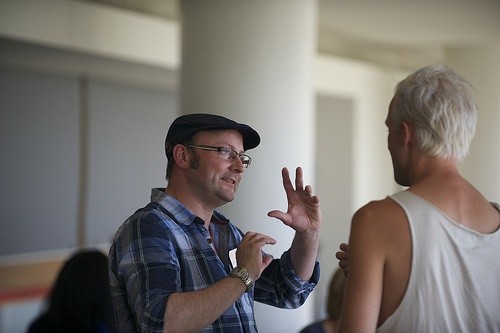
[228,266,254,293]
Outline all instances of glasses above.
[187,145,252,169]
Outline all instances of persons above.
[26,249,116,333]
[108,113,321,333]
[336,65,500,333]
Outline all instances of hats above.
[165,113,260,159]
